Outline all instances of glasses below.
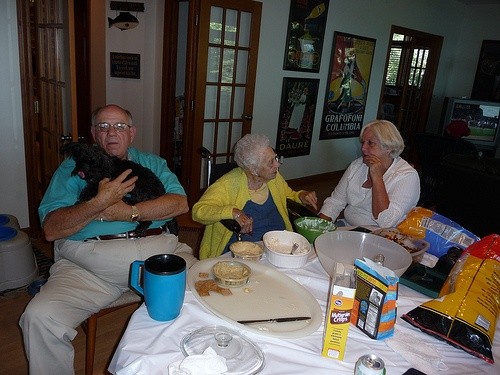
[93,121,131,134]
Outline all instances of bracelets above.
[234,210,242,219]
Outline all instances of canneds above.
[354,354,386,375]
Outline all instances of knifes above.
[237,317,311,324]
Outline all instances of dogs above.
[58,141,166,235]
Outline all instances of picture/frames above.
[470,40,500,104]
[274,77,321,159]
[283,0,331,74]
[319,30,377,141]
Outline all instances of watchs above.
[127,203,139,223]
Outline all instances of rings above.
[100,218,103,222]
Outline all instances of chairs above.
[79,288,144,375]
[415,133,478,175]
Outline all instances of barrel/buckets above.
[0,213,39,291]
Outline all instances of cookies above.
[195,272,232,296]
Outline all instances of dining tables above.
[106,240,500,375]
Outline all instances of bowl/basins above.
[369,228,431,266]
[230,241,264,260]
[294,217,337,243]
[212,260,251,288]
[315,229,412,279]
[263,231,312,267]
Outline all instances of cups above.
[130,254,187,321]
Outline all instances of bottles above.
[433,246,464,275]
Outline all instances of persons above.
[443,121,481,161]
[192,134,317,261]
[316,120,420,228]
[18,103,199,375]
[453,111,497,129]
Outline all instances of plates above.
[187,258,323,339]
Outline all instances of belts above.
[88,226,162,240]
